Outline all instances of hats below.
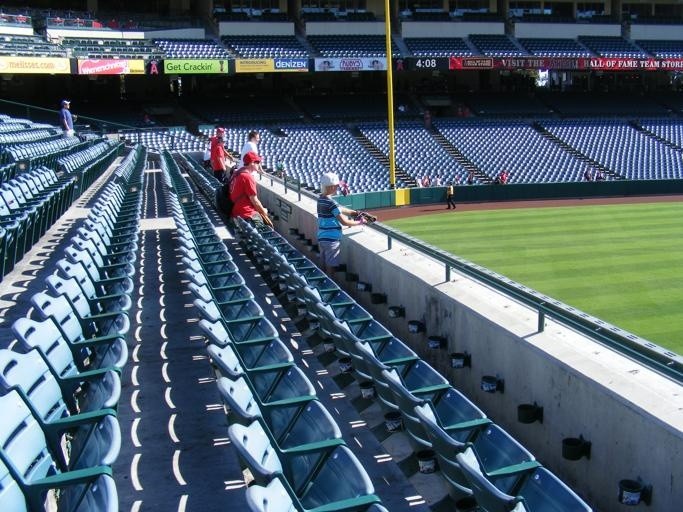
[244,152,263,165]
[322,174,340,185]
[218,137,228,142]
[217,128,225,135]
[62,101,70,105]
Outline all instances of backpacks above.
[215,171,248,220]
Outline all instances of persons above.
[342,180,350,196]
[212,139,227,183]
[446,182,456,209]
[226,152,269,220]
[424,110,431,129]
[144,114,150,124]
[317,173,368,278]
[450,102,471,118]
[60,100,74,137]
[0,7,146,30]
[211,128,235,178]
[414,170,511,188]
[240,131,267,174]
